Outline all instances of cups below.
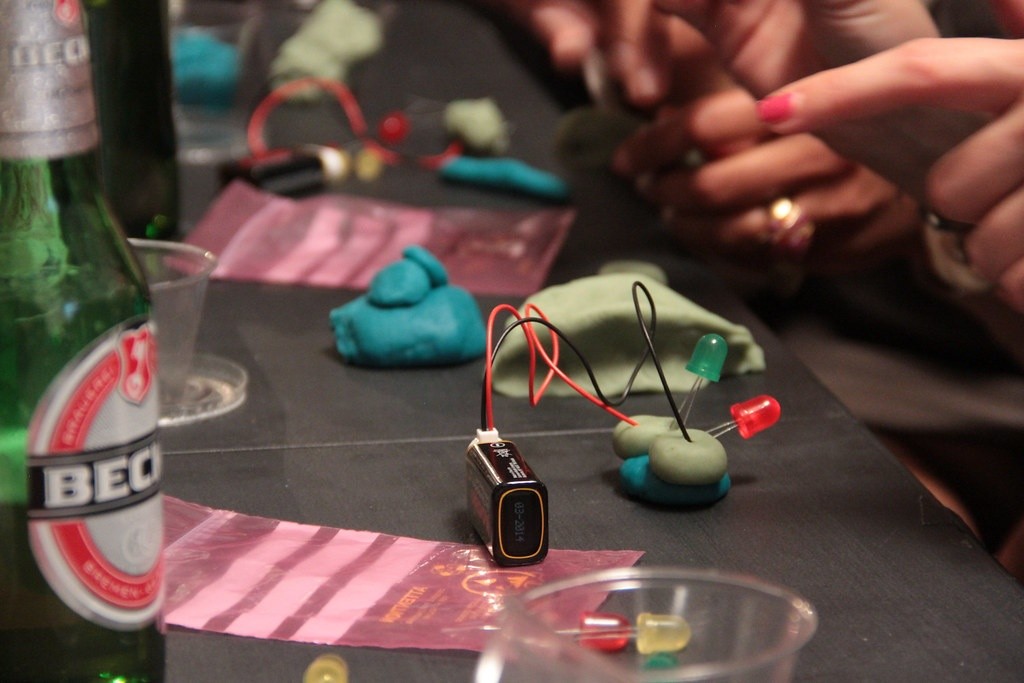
[121,237,217,408]
[479,568,816,683]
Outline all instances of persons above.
[514,1,1023,578]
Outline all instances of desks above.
[157,0,1024,683]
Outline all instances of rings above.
[769,197,815,249]
[917,186,976,233]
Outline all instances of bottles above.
[1,0,170,683]
